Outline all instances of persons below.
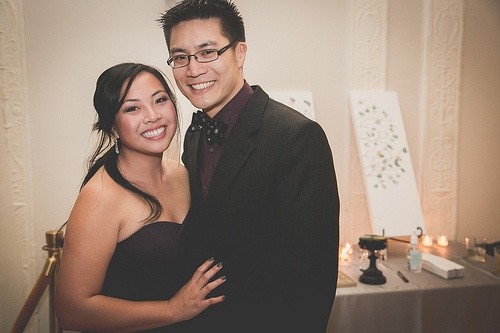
[54,63,227,333]
[157,0,339,333]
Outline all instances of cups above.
[464,236,486,263]
[405,244,422,273]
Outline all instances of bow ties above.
[189,110,227,142]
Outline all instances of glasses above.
[167,43,232,69]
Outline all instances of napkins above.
[420,253,466,280]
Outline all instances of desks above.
[326,239,500,333]
